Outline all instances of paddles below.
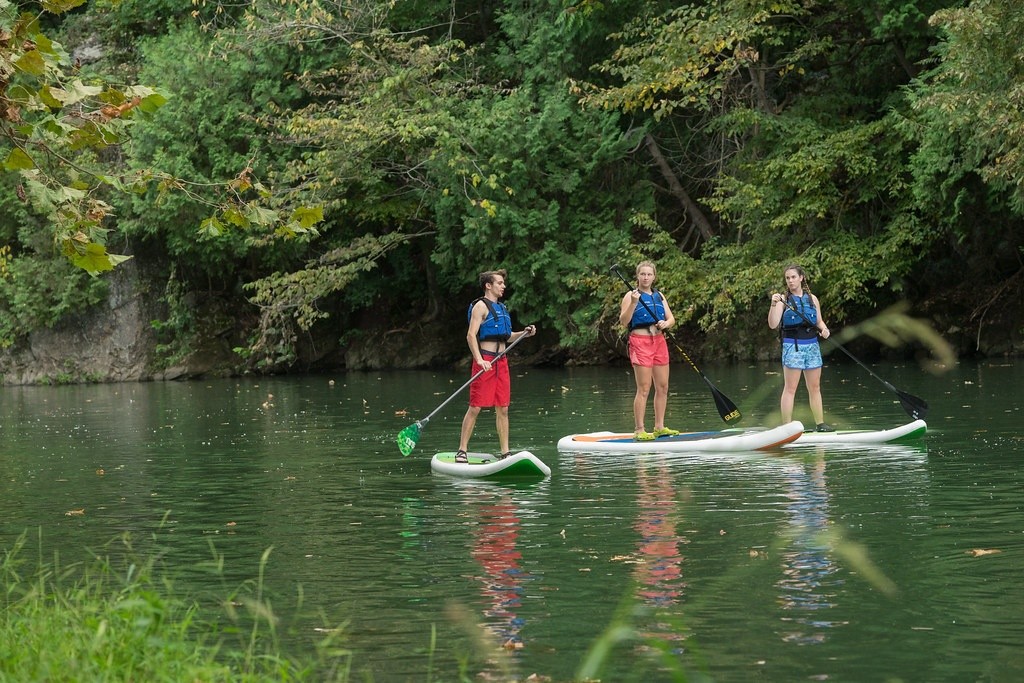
[397,325,535,457]
[610,265,742,427]
[781,297,930,421]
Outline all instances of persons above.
[619,260,680,441]
[767,266,837,432]
[454,270,536,463]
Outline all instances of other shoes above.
[803,429,813,433]
[816,423,835,432]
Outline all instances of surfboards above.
[557,419,803,455]
[430,452,552,479]
[791,417,927,445]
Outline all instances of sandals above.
[653,427,679,437]
[455,450,468,463]
[633,431,656,441]
[501,452,514,459]
